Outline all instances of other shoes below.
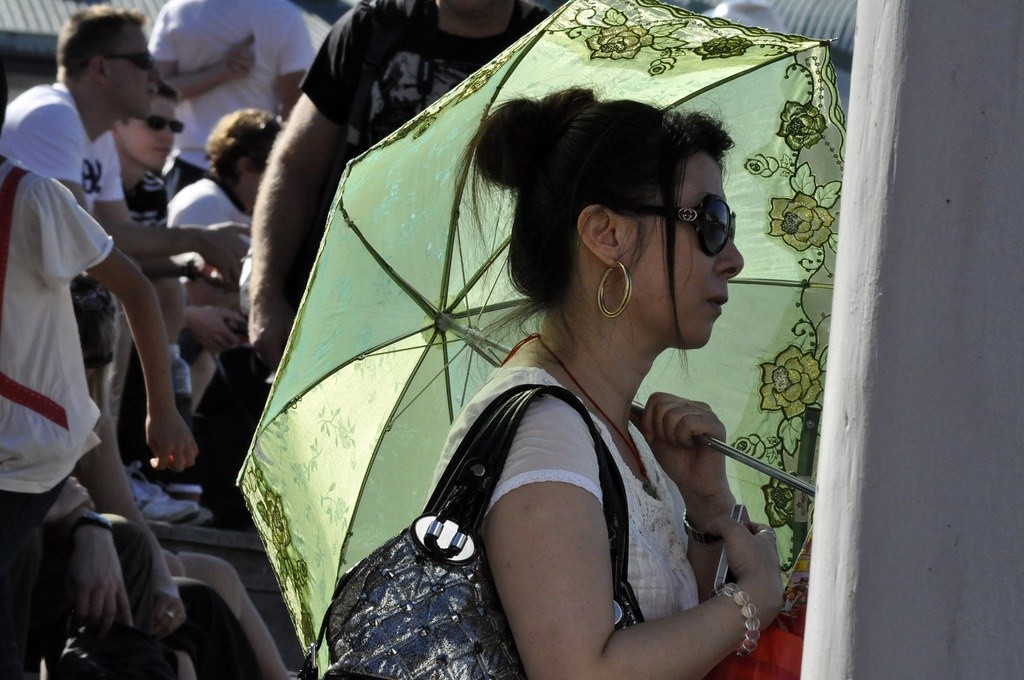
[122,462,213,526]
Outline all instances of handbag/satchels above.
[297,384,646,680]
[56,617,178,680]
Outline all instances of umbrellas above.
[235,0,848,680]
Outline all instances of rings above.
[760,529,777,538]
[165,610,177,624]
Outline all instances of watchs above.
[66,511,114,554]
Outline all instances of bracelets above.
[683,507,725,546]
[710,583,761,657]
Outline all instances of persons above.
[423,86,783,680]
[1,1,552,680]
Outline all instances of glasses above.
[617,194,736,257]
[258,120,280,138]
[132,115,184,133]
[83,53,155,71]
[84,352,113,369]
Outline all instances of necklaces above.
[500,333,656,496]
